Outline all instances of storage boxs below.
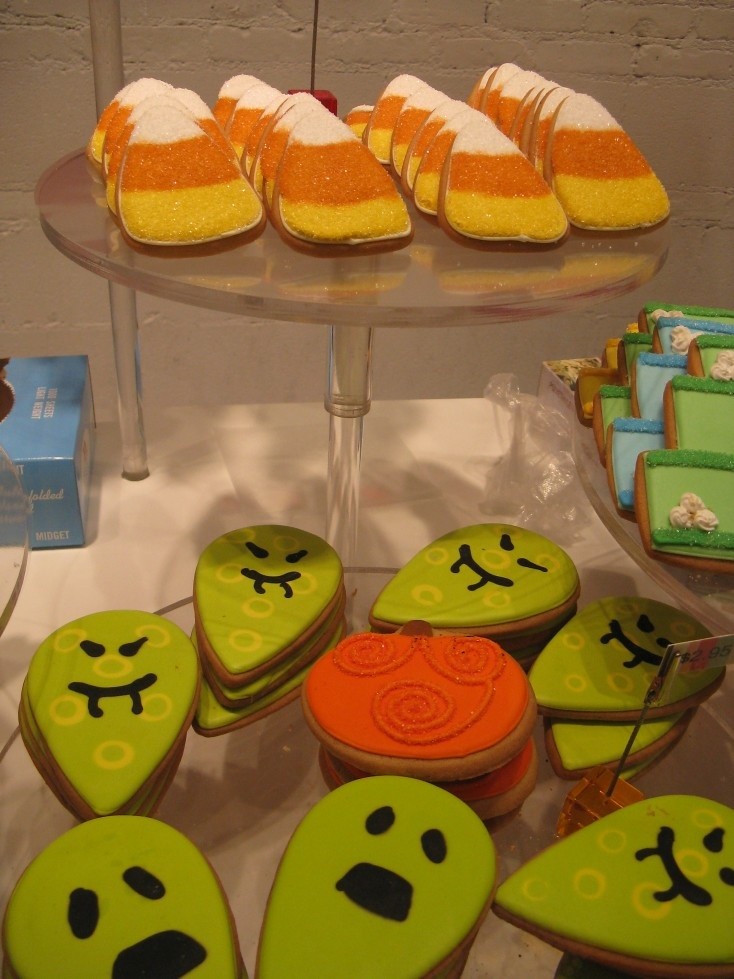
[1,354,99,553]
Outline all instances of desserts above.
[576,302,734,565]
[84,57,672,254]
[2,523,732,979]
